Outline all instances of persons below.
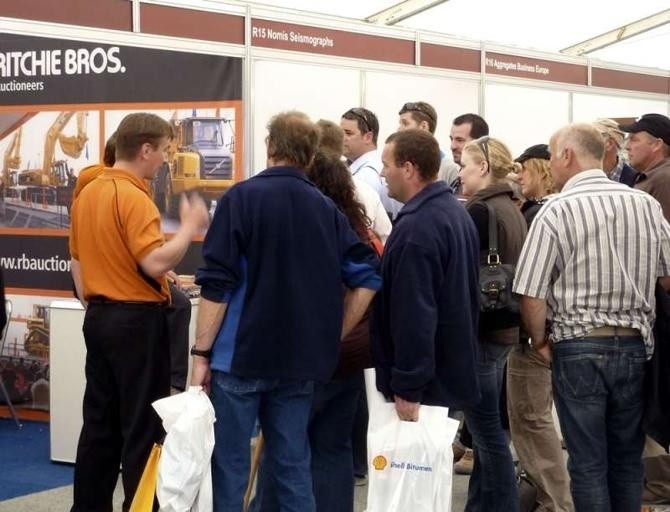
[69,101,670,512]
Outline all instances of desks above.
[49,298,200,471]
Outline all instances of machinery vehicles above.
[18,108,89,206]
[0,125,23,198]
[147,106,236,221]
[0,302,48,359]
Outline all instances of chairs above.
[1,300,24,428]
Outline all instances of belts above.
[584,326,641,337]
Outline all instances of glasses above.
[477,136,490,173]
[351,108,371,132]
[405,103,434,121]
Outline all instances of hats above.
[591,119,625,150]
[514,144,550,162]
[618,114,670,146]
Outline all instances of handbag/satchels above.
[477,201,515,313]
[640,454,670,504]
[515,472,538,512]
[366,402,461,512]
[128,385,217,512]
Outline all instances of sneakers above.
[454,447,475,475]
